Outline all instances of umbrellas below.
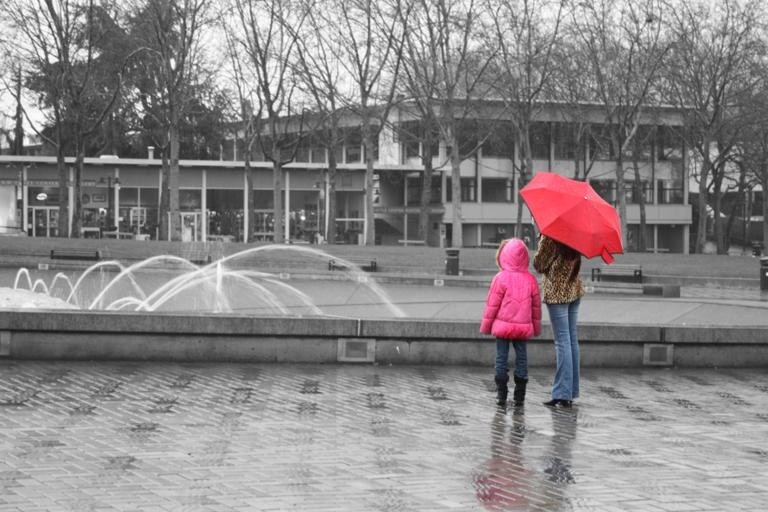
[518,170,625,264]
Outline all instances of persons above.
[95,211,105,238]
[533,407,582,512]
[533,231,584,409]
[480,238,544,409]
[471,404,534,512]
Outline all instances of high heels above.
[543,399,572,408]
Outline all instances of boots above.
[513,384,526,401]
[494,386,507,405]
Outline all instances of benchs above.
[50,246,100,260]
[591,264,642,282]
[163,250,213,265]
[328,256,377,272]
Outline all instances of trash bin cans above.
[446,250,459,275]
[760,259,768,290]
